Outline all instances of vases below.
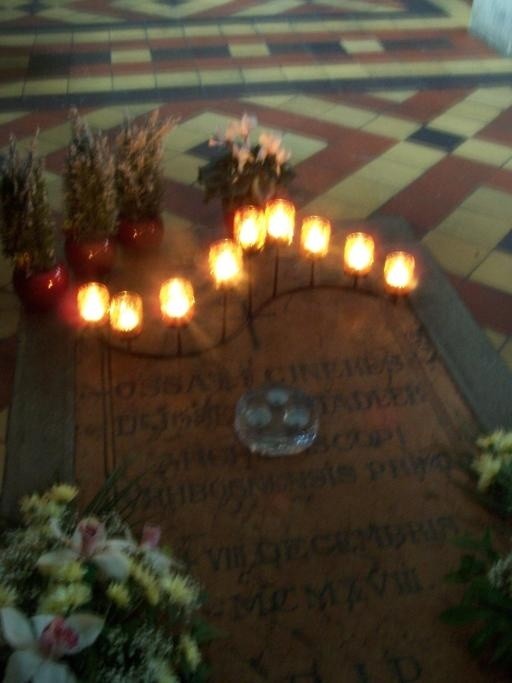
[64,235,119,282]
[225,202,263,239]
[13,265,67,309]
[115,215,166,256]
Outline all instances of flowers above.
[437,425,512,520]
[0,455,216,682]
[197,111,296,215]
[1,124,60,266]
[61,102,120,238]
[111,109,183,219]
